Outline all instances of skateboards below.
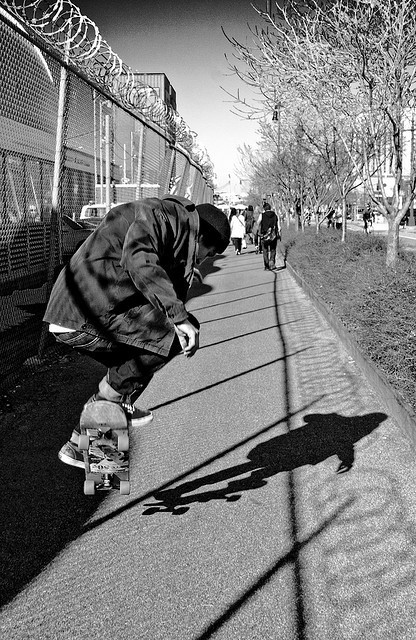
[78,400,129,496]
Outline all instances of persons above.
[42,194,231,474]
[257,203,281,271]
[304,204,343,229]
[229,205,262,255]
[363,204,375,229]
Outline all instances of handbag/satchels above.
[242,240,246,249]
[261,215,277,245]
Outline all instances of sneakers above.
[57,439,86,470]
[83,393,154,427]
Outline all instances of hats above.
[196,203,230,241]
